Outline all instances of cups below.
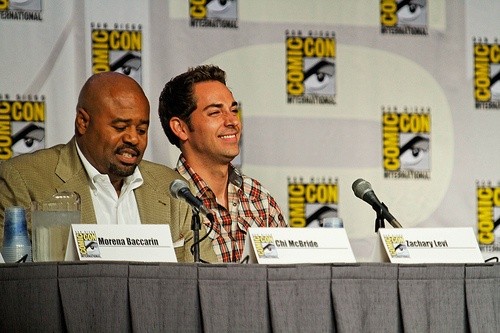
[323,218,344,229]
[1,207,33,263]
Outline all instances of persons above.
[0,72,217,265]
[155,64,287,266]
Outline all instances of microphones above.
[169,179,214,222]
[353,178,402,229]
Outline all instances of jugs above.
[29,190,81,262]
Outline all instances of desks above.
[0,263,500,333]
